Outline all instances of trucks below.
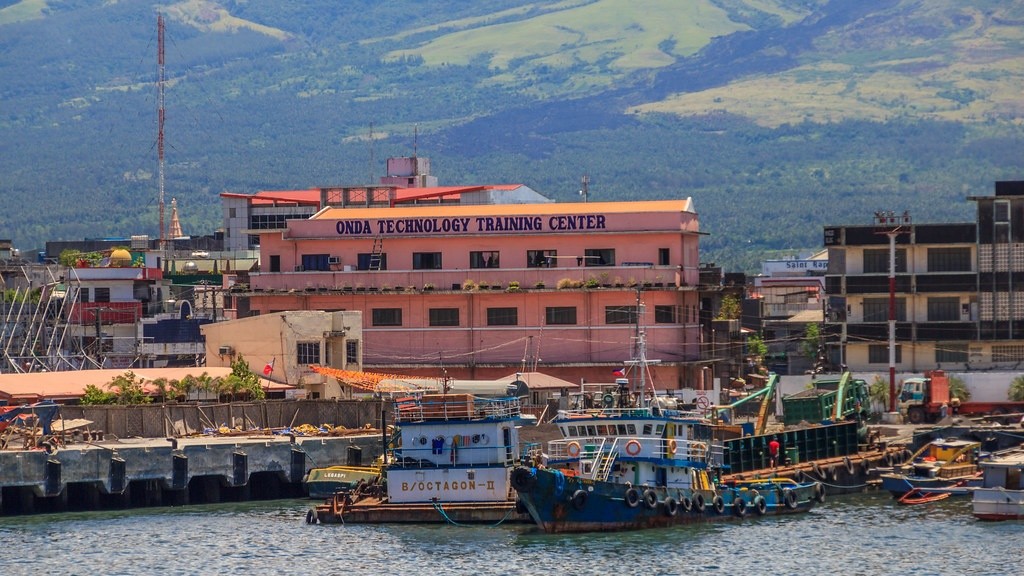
[781,379,873,430]
[897,378,1024,425]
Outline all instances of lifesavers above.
[624,488,640,508]
[566,441,582,457]
[604,394,614,404]
[516,496,522,515]
[625,440,641,456]
[692,492,706,512]
[794,464,838,503]
[681,496,691,510]
[669,439,678,454]
[755,495,768,515]
[844,456,855,475]
[306,508,317,524]
[861,458,870,475]
[712,495,725,514]
[883,449,914,466]
[572,490,590,511]
[643,489,659,510]
[734,497,747,516]
[509,467,537,493]
[664,497,679,516]
[693,443,706,456]
[785,488,799,509]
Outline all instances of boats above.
[306,454,398,500]
[509,301,826,533]
[719,419,912,497]
[878,437,1024,522]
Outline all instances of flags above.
[612,367,625,377]
[264,362,273,375]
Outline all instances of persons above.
[940,401,948,418]
[768,438,779,470]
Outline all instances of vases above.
[668,283,675,287]
[681,282,688,286]
[231,287,416,293]
[452,284,460,290]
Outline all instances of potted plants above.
[463,272,664,291]
[423,282,434,291]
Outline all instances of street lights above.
[872,210,913,413]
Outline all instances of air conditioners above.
[328,256,340,264]
